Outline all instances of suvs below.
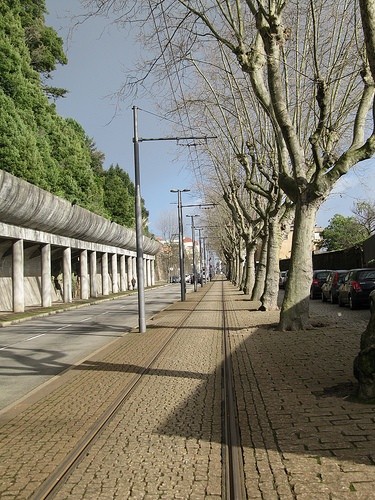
[321,270,349,304]
[306,269,332,299]
[338,268,375,310]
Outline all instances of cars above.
[168,270,209,284]
[277,269,290,289]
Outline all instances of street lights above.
[186,214,201,271]
[170,188,190,301]
